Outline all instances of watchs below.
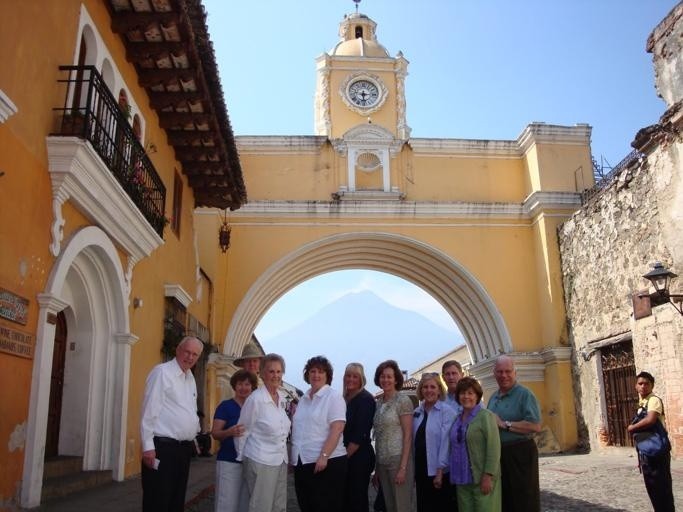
[505,419,512,433]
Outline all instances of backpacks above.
[629,394,674,463]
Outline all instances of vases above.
[62,113,93,141]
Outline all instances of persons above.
[486,355,543,512]
[342,363,376,512]
[628,371,677,512]
[291,355,349,512]
[373,360,417,511]
[443,360,486,417]
[411,372,458,512]
[211,342,300,512]
[140,335,204,512]
[447,376,503,512]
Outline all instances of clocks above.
[338,71,389,116]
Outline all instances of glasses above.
[421,371,440,380]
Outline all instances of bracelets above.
[400,465,408,471]
[320,453,329,458]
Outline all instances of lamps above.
[642,260,683,316]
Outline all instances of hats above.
[232,343,266,368]
